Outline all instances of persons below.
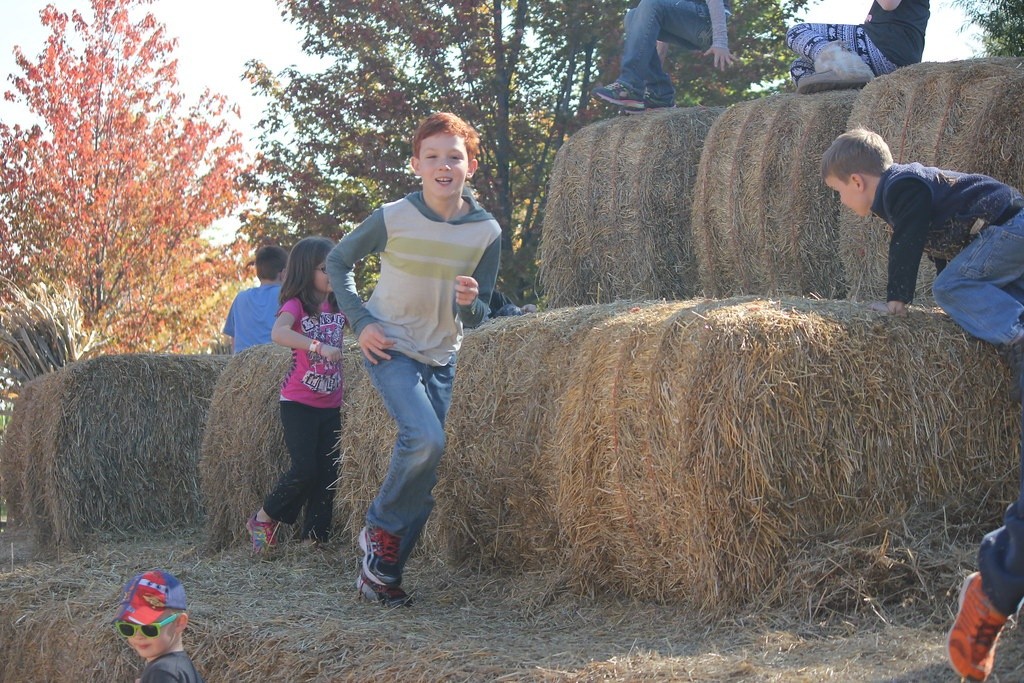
[820,127,1024,406]
[785,0,932,94]
[327,110,502,614]
[245,237,352,558]
[220,246,289,356]
[593,0,741,115]
[944,407,1024,683]
[110,569,204,683]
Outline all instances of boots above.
[799,39,875,93]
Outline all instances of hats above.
[111,571,186,626]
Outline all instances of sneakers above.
[356,569,413,609]
[592,81,645,110]
[621,98,676,114]
[359,525,402,589]
[945,571,1008,683]
[246,514,280,560]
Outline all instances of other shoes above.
[997,334,1024,404]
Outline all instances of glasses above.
[313,266,327,274]
[116,613,181,639]
